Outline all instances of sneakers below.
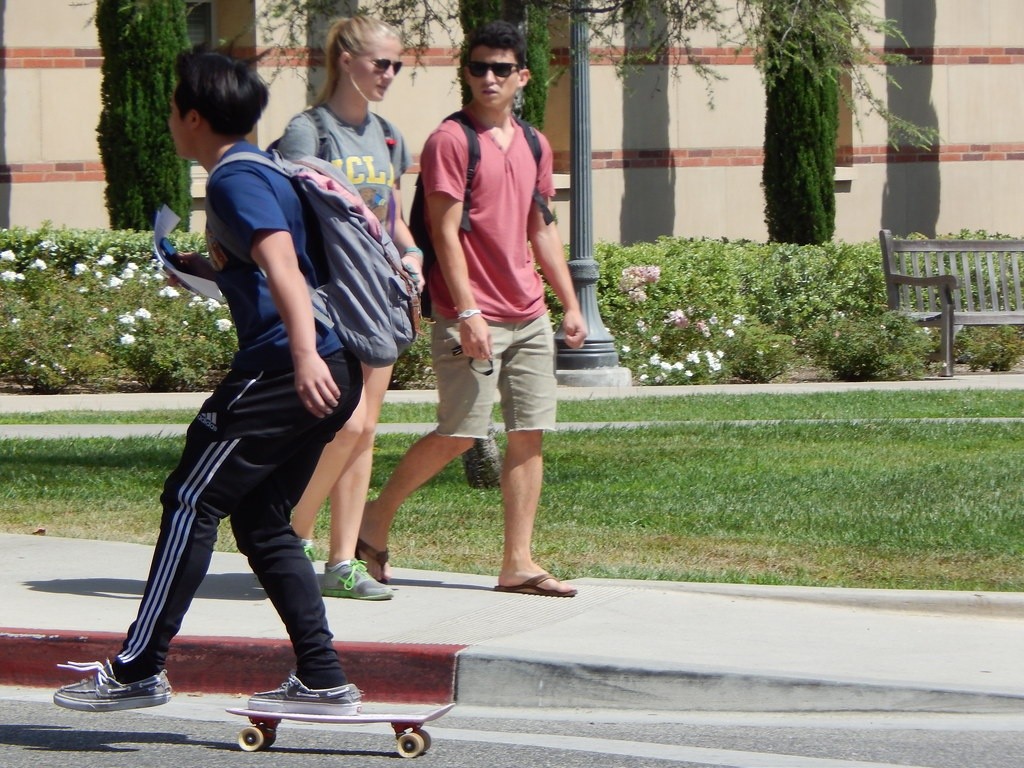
[299,538,316,565]
[53,656,172,712]
[321,560,396,600]
[247,673,361,717]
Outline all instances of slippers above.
[354,537,391,586]
[494,574,578,597]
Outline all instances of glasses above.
[369,58,403,77]
[464,61,522,78]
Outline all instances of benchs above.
[879,228,1024,377]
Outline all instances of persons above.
[278,17,427,602]
[53,53,366,711]
[353,19,590,597]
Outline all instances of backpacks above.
[204,148,425,367]
[265,110,396,163]
[407,111,556,320]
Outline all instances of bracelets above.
[457,309,479,321]
[403,247,424,257]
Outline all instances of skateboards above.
[225,702,456,758]
